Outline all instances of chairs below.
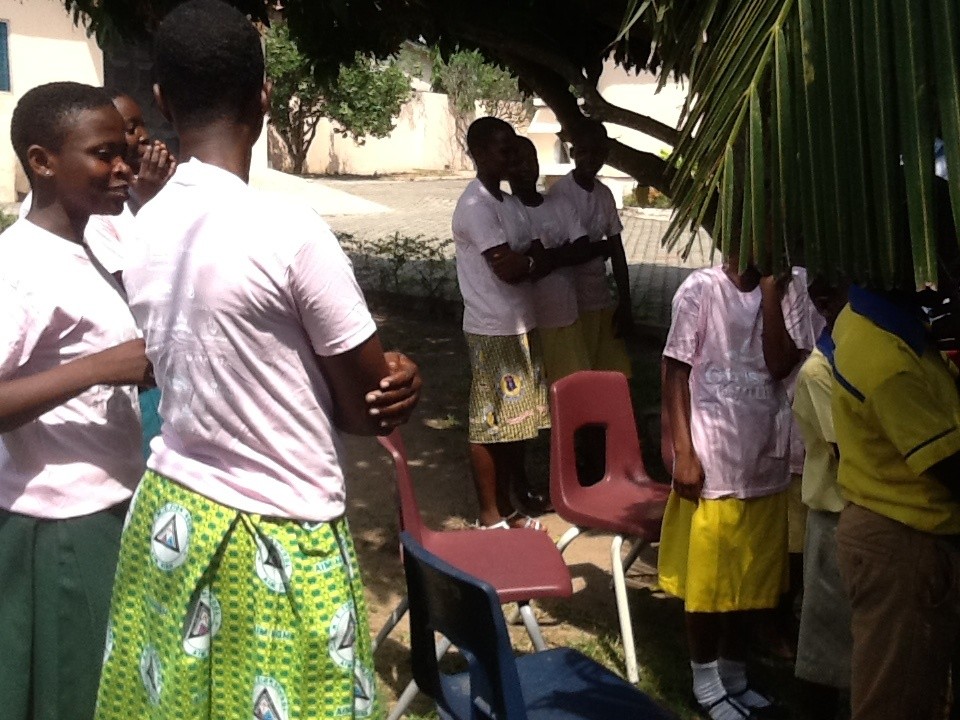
[372,428,572,720]
[399,530,674,720]
[552,370,679,684]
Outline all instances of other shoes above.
[690,690,756,720]
[728,676,798,720]
[761,645,796,666]
[527,490,556,512]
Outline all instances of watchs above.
[527,255,534,272]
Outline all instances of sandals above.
[501,510,549,535]
[475,518,513,530]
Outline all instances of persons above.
[0,82,188,719]
[541,121,645,478]
[1,84,175,468]
[449,117,560,534]
[651,183,960,720]
[512,138,599,511]
[95,0,422,720]
